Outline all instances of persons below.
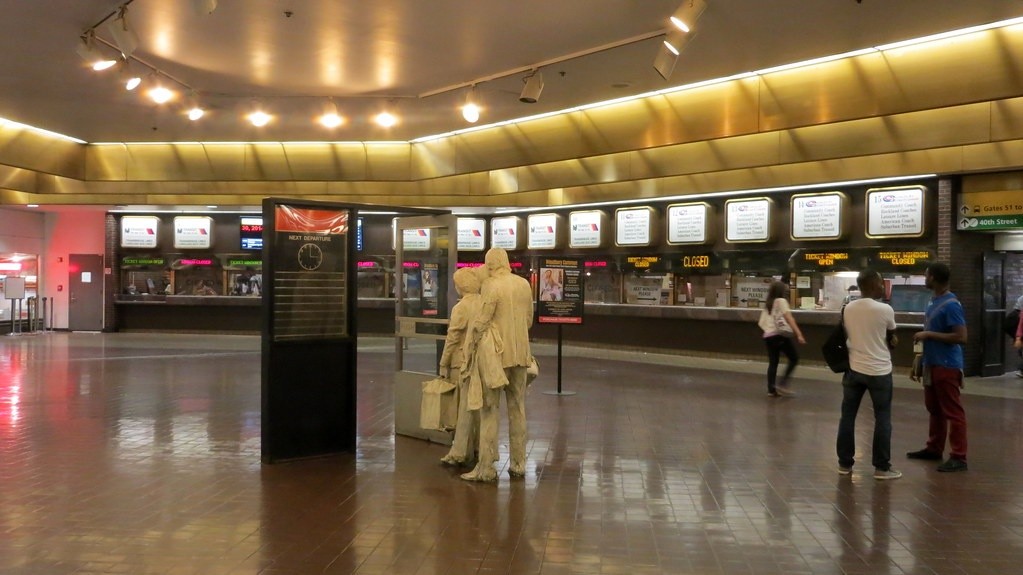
[540,268,563,301]
[843,285,859,301]
[422,270,433,297]
[199,279,217,295]
[758,282,808,397]
[836,269,902,479]
[161,277,172,293]
[1013,295,1023,379]
[239,272,262,295]
[906,263,967,472]
[439,247,533,481]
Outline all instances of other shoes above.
[873,469,901,479]
[838,464,853,474]
[767,389,782,396]
[774,384,796,395]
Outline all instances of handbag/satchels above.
[910,354,923,383]
[773,313,797,338]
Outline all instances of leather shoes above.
[906,448,944,462]
[937,457,968,472]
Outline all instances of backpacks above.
[822,304,850,374]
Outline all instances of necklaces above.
[941,289,947,295]
